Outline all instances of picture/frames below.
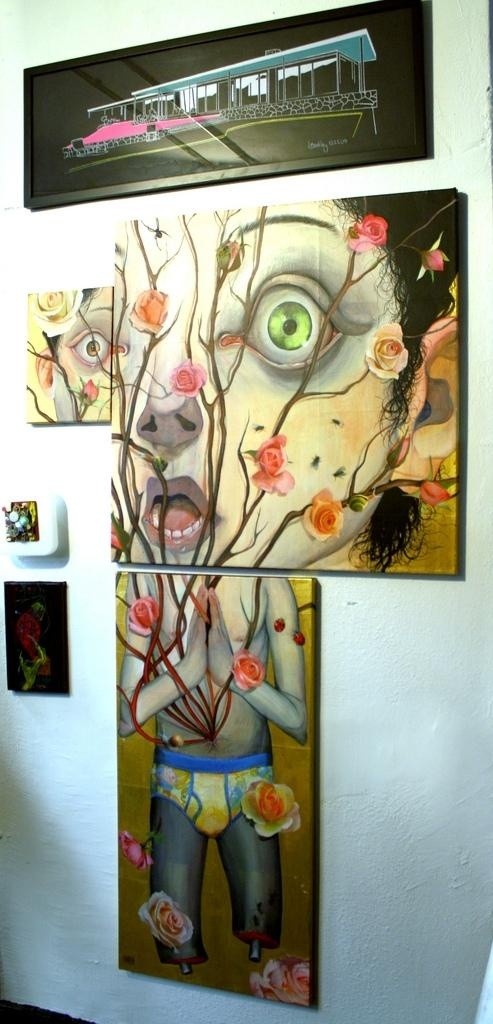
[23,0,432,213]
